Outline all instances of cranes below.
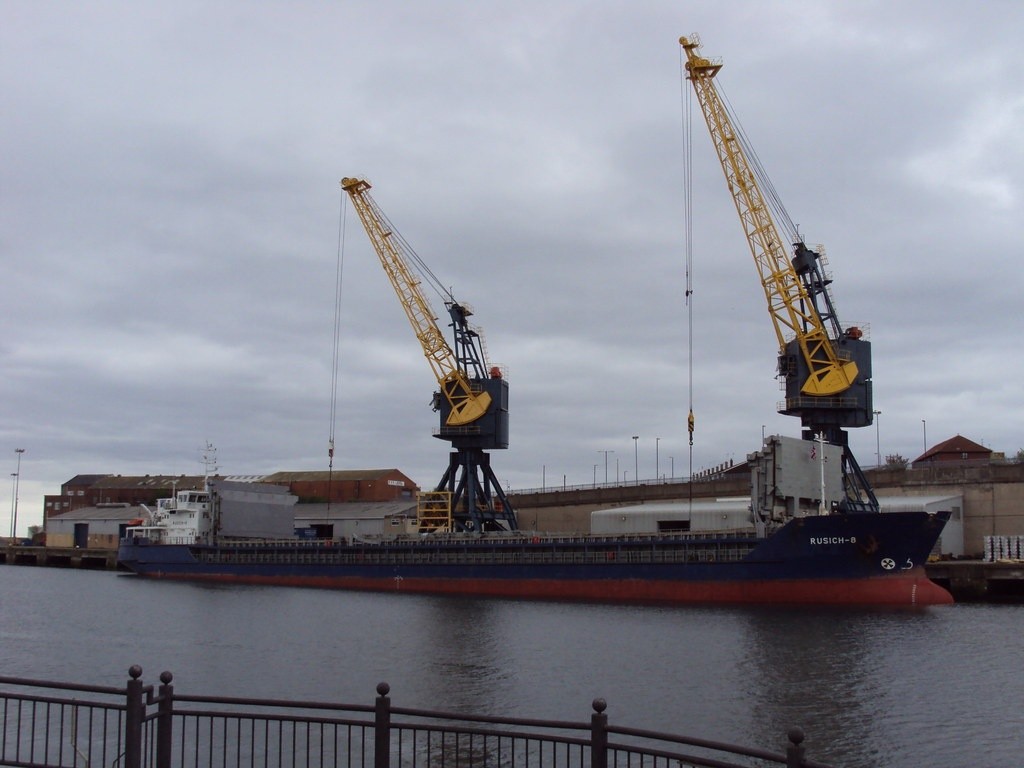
[326,173,519,532]
[679,32,881,519]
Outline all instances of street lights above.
[873,409,881,467]
[762,425,766,446]
[656,438,661,484]
[922,420,926,455]
[15,447,25,544]
[669,457,673,478]
[624,471,628,481]
[9,473,18,544]
[632,436,639,486]
[597,451,614,490]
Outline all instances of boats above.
[115,438,954,612]
